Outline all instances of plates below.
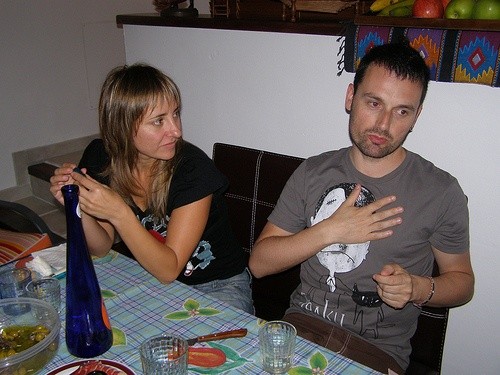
[43,358,137,375]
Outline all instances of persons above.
[249,42,474,375]
[50,64,256,317]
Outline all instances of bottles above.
[61,184,113,359]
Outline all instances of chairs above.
[0,200,65,266]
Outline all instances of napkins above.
[31,243,66,273]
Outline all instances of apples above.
[411,0,500,19]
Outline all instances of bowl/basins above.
[0,297,60,375]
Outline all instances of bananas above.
[370,0,414,16]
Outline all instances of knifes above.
[186,329,247,346]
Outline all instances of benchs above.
[211,142,450,375]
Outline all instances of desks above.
[0,248,385,375]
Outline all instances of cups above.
[0,267,34,316]
[257,321,297,375]
[139,334,188,375]
[25,278,61,320]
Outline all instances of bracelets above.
[412,275,436,307]
